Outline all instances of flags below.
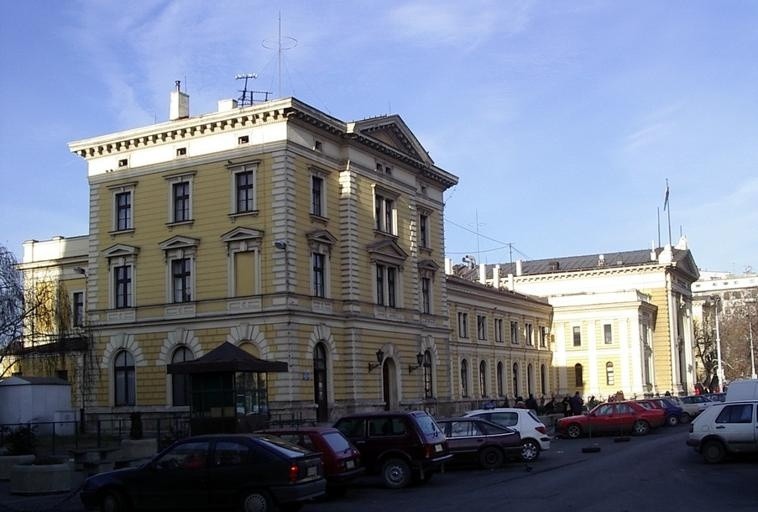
[665,188,669,209]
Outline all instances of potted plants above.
[0,410,159,496]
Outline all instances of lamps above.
[407,351,424,374]
[368,348,385,371]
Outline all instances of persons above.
[562,393,573,417]
[513,397,526,408]
[525,393,538,410]
[572,391,583,415]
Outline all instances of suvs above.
[684,399,757,464]
[445,407,552,464]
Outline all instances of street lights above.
[745,302,757,379]
[712,295,725,394]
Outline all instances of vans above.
[726,380,757,403]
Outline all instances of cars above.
[250,426,362,494]
[80,433,329,509]
[436,416,524,468]
[555,400,667,438]
[331,409,451,490]
[637,393,726,428]
